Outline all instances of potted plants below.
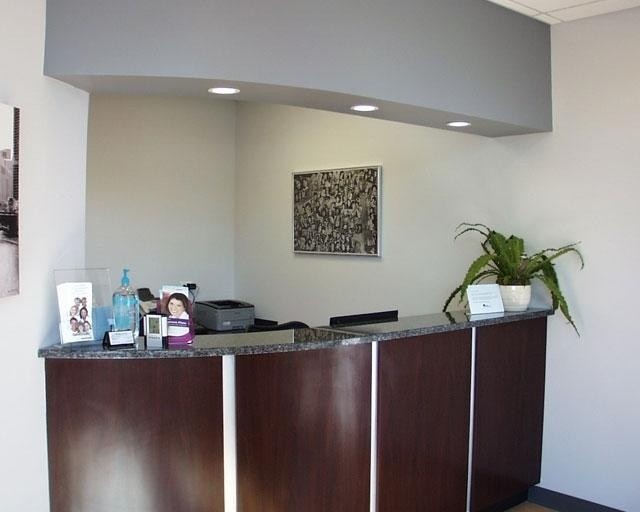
[441,222,585,340]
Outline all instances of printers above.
[194,298,256,331]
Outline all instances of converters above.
[187,283,197,292]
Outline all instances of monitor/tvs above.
[330,309,399,328]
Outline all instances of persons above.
[70,297,92,335]
[167,293,190,337]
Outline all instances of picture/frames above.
[292,165,383,259]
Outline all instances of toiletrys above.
[112,267,140,340]
